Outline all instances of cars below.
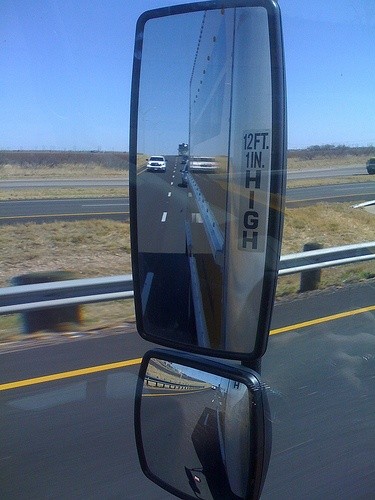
[180,162,189,186]
[146,156,166,172]
[190,157,218,173]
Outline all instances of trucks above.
[178,143,188,158]
